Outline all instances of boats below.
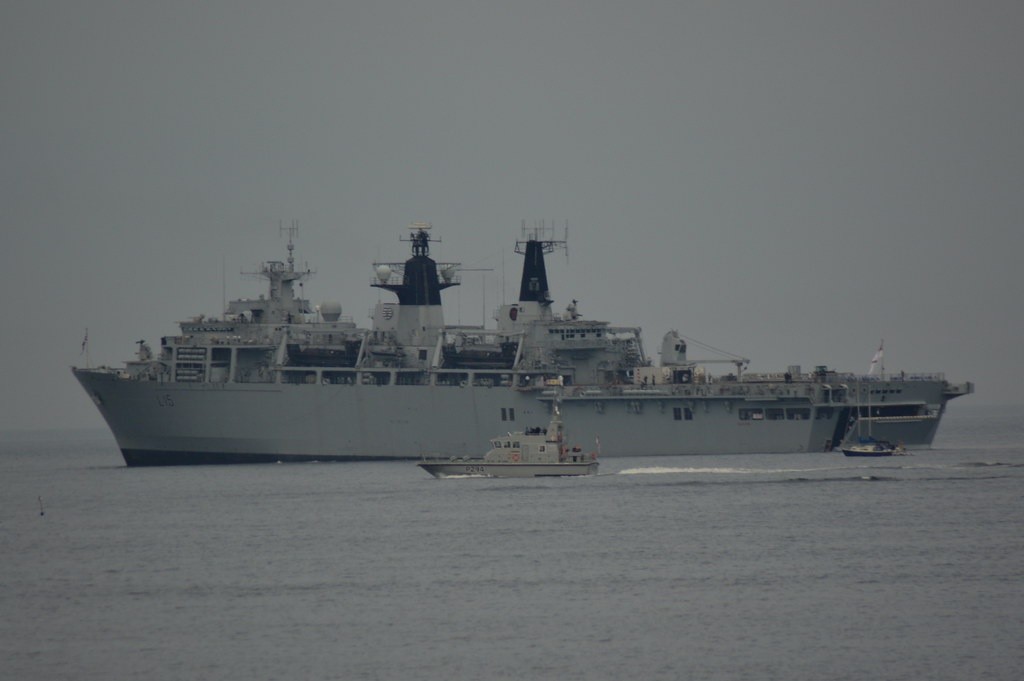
[841,385,906,457]
[416,419,601,478]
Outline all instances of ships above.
[71,215,976,466]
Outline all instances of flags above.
[872,341,882,362]
[80,331,88,354]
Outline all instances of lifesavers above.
[513,453,520,462]
